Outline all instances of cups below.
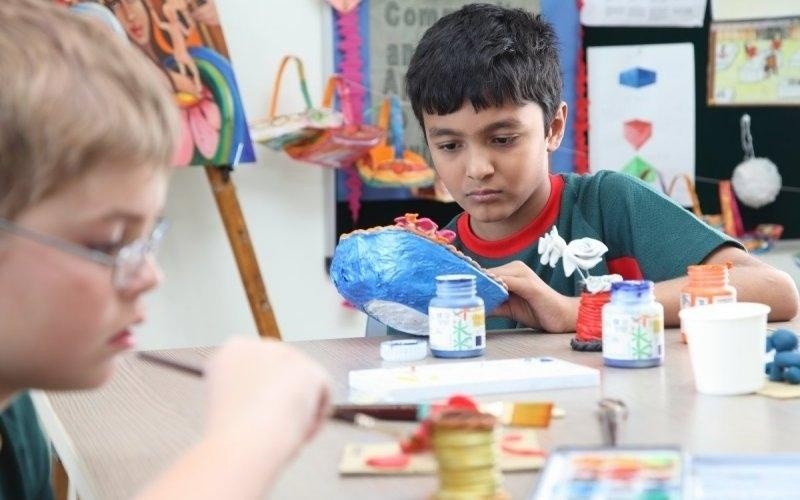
[683,299,772,396]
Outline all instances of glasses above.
[1,217,171,289]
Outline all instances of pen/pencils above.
[336,402,428,425]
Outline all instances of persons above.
[386,3,800,336]
[1,0,332,500]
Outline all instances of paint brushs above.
[134,352,377,437]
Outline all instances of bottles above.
[599,280,664,369]
[681,266,736,343]
[417,408,505,500]
[428,274,487,358]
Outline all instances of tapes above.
[381,338,429,361]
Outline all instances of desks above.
[24,324,800,500]
